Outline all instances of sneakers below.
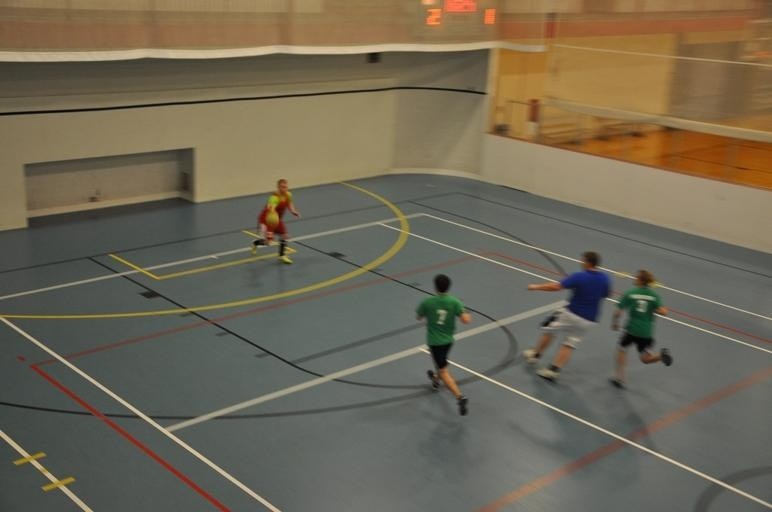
[428,369,440,390]
[660,347,672,366]
[278,254,293,264]
[250,241,258,255]
[535,367,557,380]
[457,395,467,416]
[609,375,625,387]
[523,349,539,363]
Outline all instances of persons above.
[248,178,302,264]
[413,274,473,417]
[608,268,673,390]
[521,250,611,382]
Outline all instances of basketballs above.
[266,213,279,225]
